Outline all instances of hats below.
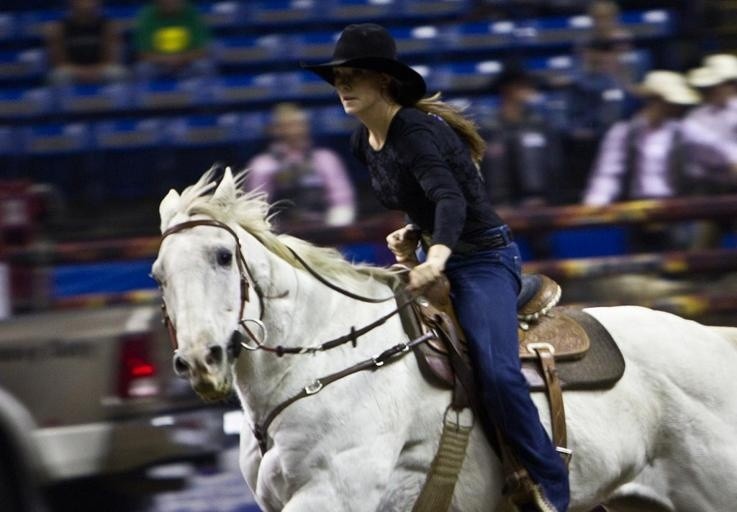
[302,21,427,107]
[624,69,704,106]
[687,54,737,89]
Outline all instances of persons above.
[0,149,72,316]
[296,20,571,512]
[574,0,635,82]
[579,66,737,210]
[670,53,737,204]
[240,101,357,234]
[131,0,213,76]
[42,1,136,84]
[467,65,563,214]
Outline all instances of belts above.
[419,230,514,256]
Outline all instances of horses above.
[150,161,737,512]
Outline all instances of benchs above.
[2,2,736,307]
